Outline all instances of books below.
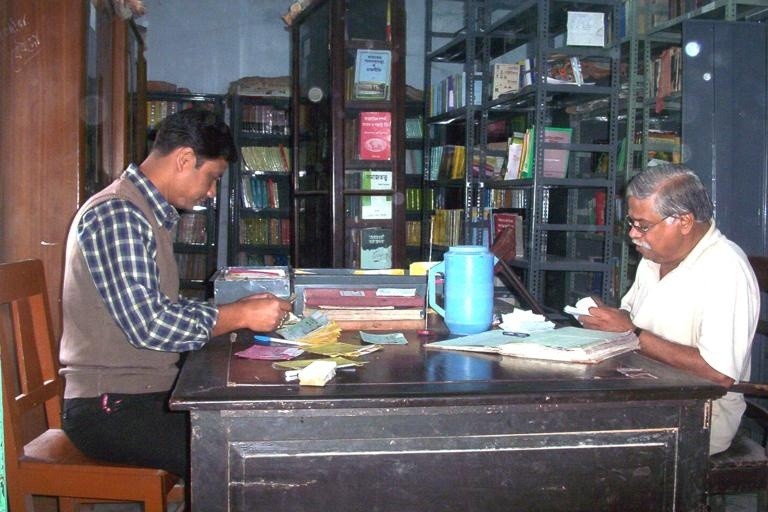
[145,78,295,298]
[405,46,687,300]
[422,325,641,365]
[302,287,427,333]
[298,18,404,275]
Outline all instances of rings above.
[283,311,289,321]
[278,319,285,329]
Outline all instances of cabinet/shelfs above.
[578,3,766,288]
[2,1,149,355]
[425,0,624,310]
[286,1,408,270]
[148,77,290,300]
[406,85,423,262]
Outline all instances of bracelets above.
[632,327,642,336]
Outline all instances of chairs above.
[0,258,190,512]
[700,257,767,512]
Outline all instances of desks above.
[164,296,726,508]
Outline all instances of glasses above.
[623,212,676,233]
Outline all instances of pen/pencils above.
[253,336,308,346]
[503,332,530,336]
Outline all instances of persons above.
[56,107,293,510]
[577,163,761,456]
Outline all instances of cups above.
[426,243,502,337]
